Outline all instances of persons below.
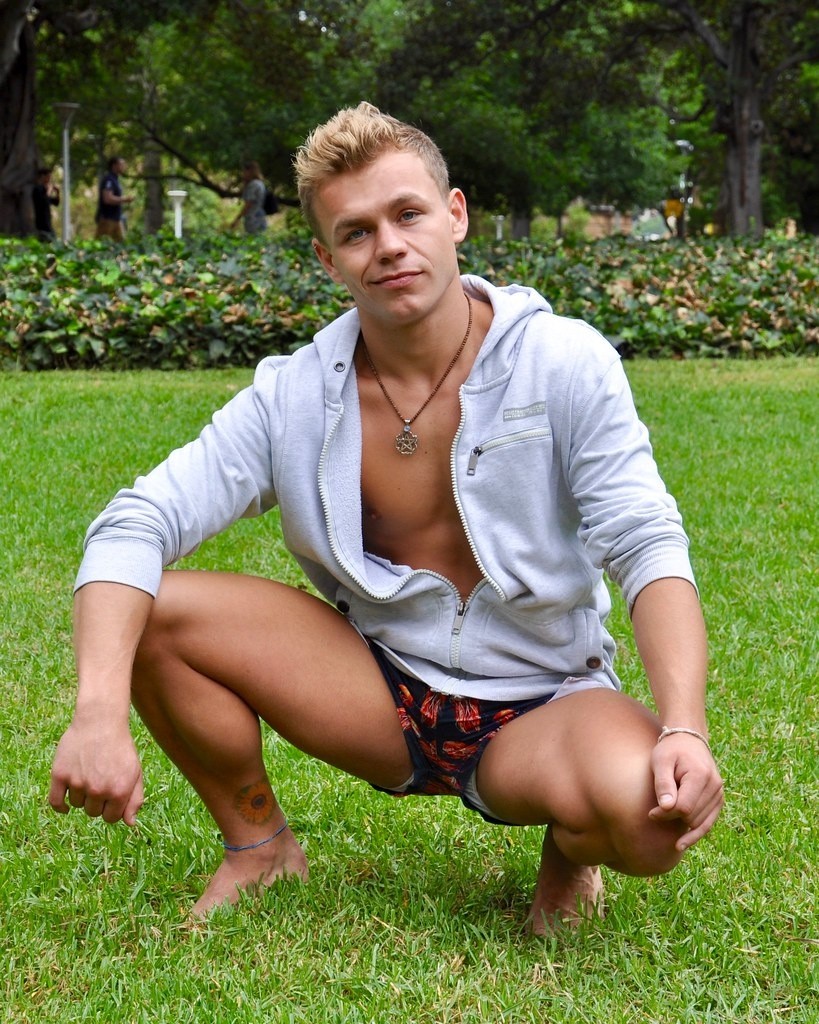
[230,159,268,237]
[46,98,726,945]
[95,155,135,245]
[31,166,60,243]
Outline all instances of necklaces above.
[360,288,475,457]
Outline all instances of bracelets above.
[220,817,290,853]
[654,725,711,758]
